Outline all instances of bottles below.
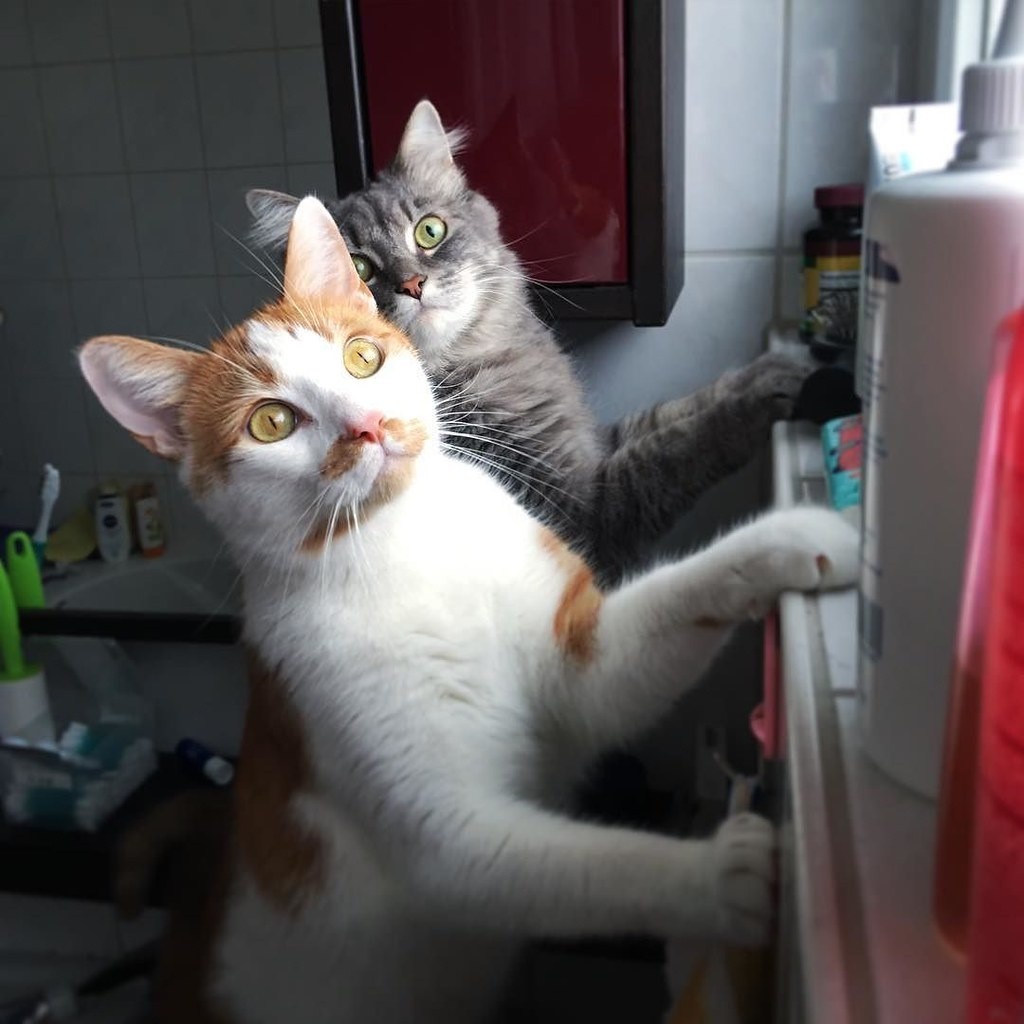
[784,37,1019,1019]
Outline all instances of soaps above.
[170,537,203,559]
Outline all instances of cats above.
[242,98,812,580]
[67,195,859,1024]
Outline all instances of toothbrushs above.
[27,463,64,576]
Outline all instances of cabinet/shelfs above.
[719,322,1024,1019]
[317,0,688,328]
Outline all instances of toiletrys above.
[852,98,964,413]
[842,49,1024,806]
[92,478,133,565]
[133,475,171,558]
[818,406,865,527]
[925,287,1023,1024]
